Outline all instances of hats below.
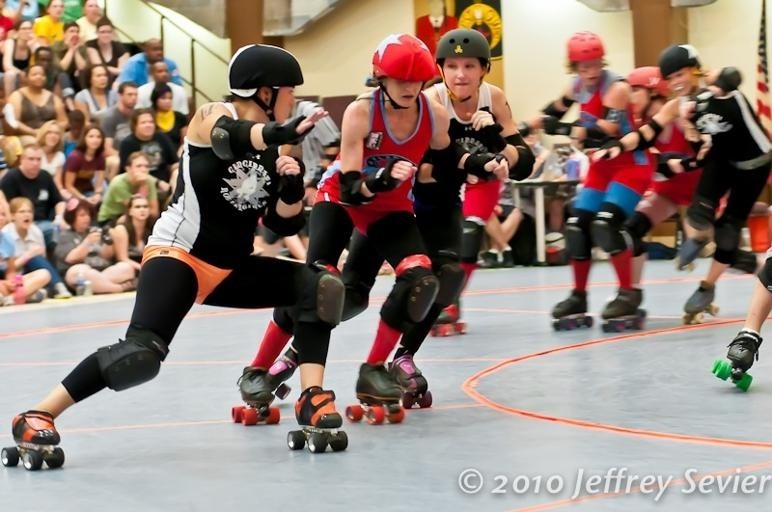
[150,83,171,104]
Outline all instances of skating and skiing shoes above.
[287,386,348,453]
[388,354,432,408]
[1,410,65,471]
[431,303,467,337]
[683,281,719,324]
[232,367,280,426]
[711,331,762,392]
[346,363,404,424]
[551,288,592,331]
[675,237,717,272]
[602,287,647,332]
[265,354,298,400]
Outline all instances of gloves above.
[464,152,510,181]
[365,157,415,193]
[684,87,716,114]
[262,115,315,147]
[591,139,624,162]
[276,156,305,205]
[478,106,506,154]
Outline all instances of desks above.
[503,174,580,265]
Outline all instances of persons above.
[237,31,511,408]
[264,27,541,393]
[0,0,772,327]
[9,43,347,448]
[724,249,772,373]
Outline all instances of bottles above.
[73,270,85,296]
[13,274,26,304]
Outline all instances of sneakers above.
[117,278,138,292]
[25,288,48,303]
[478,251,499,268]
[501,250,514,267]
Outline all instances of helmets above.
[660,43,700,80]
[568,31,604,62]
[372,33,436,82]
[625,66,671,98]
[436,28,492,64]
[227,44,303,99]
